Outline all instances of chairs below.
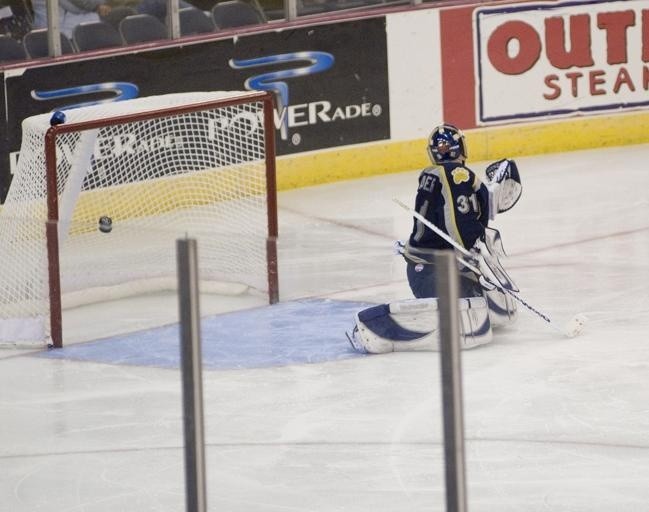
[0,0,265,64]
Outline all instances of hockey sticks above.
[390,197,587,338]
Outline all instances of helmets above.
[427,124,467,164]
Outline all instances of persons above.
[345,123,524,355]
[0,0,196,43]
